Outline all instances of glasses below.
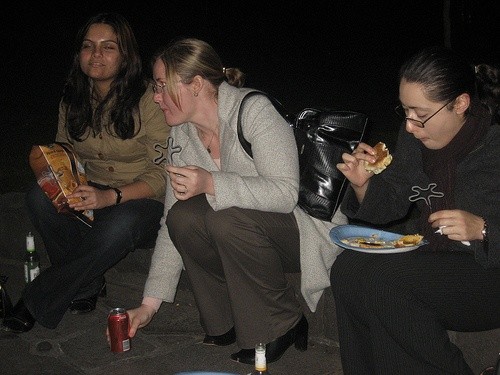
[151,74,194,95]
[393,93,456,128]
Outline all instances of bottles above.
[23,231,42,283]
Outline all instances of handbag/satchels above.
[237,91,370,222]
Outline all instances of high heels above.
[70,271,106,315]
[2,296,35,334]
[232,314,308,363]
[202,324,235,346]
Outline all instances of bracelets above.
[109,186,123,204]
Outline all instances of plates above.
[330,223,429,254]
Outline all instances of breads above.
[392,234,424,248]
[365,141,393,175]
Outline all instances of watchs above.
[479,215,489,243]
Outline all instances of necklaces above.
[198,124,218,153]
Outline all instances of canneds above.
[107,307,131,353]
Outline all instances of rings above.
[185,184,189,194]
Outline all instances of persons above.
[104,39,349,366]
[330,48,499,375]
[0,14,172,331]
